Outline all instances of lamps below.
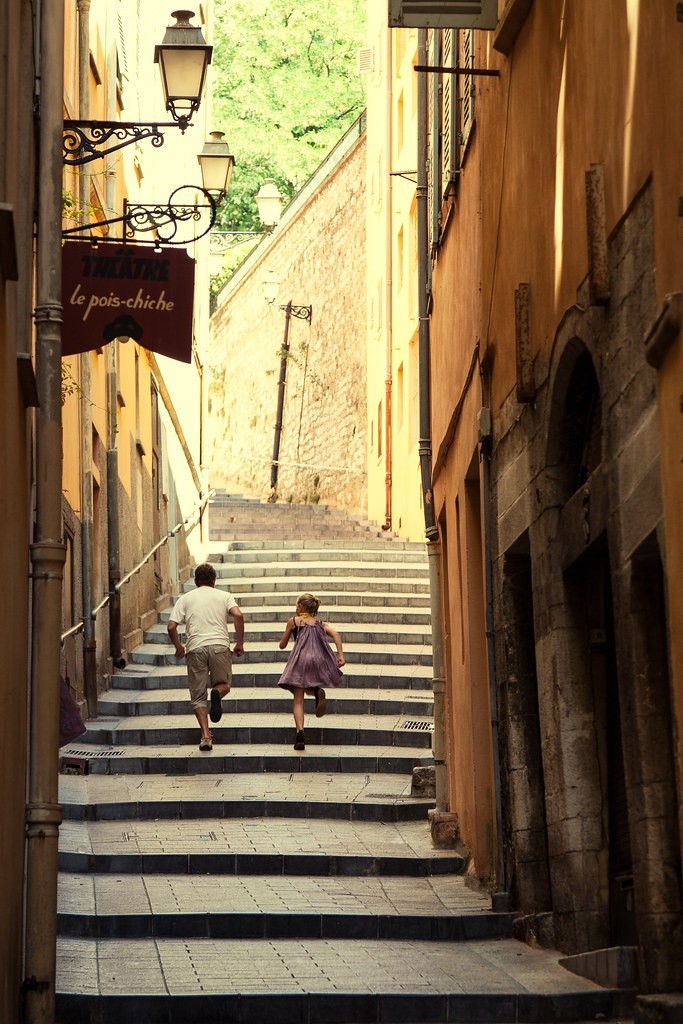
[154,10,213,136]
[254,178,284,236]
[197,132,236,207]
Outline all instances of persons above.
[279,592,345,751]
[167,563,244,752]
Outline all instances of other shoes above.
[314,686,326,718]
[198,737,212,751]
[294,730,306,750]
[210,688,223,723]
